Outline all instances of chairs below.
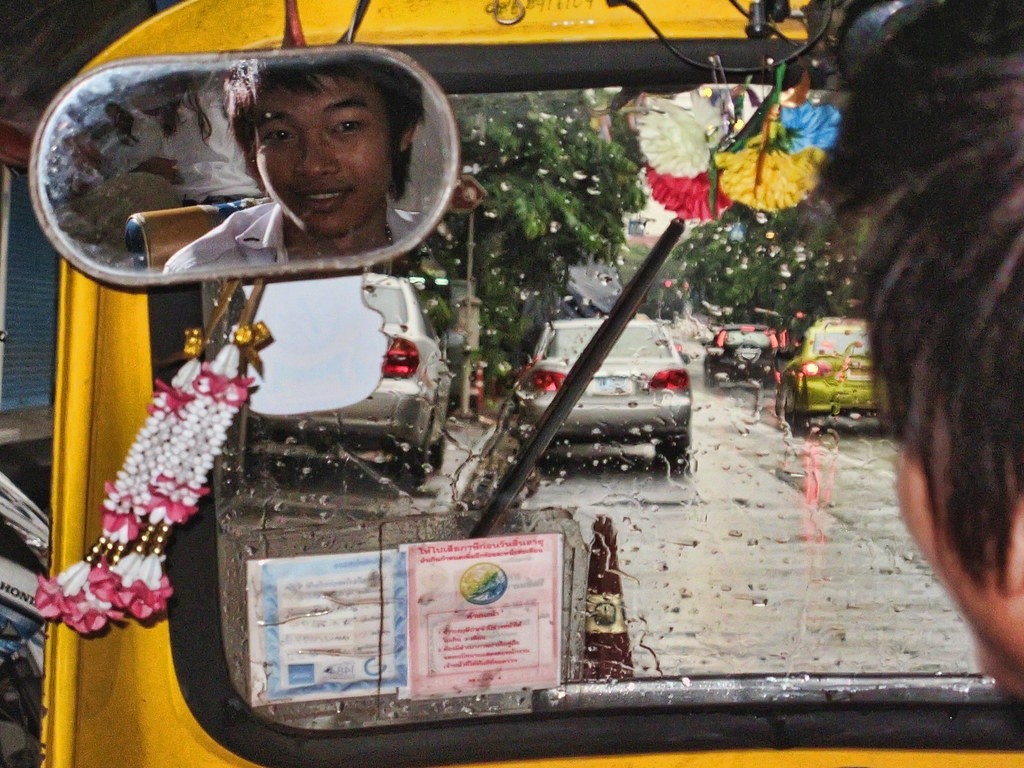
[123,199,270,276]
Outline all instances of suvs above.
[703,323,775,390]
[247,273,453,474]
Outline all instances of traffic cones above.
[575,515,634,681]
[473,366,486,412]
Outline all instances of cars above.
[505,317,691,458]
[775,314,876,425]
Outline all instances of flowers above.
[629,64,843,226]
[32,360,255,636]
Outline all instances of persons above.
[803,1,1024,697]
[162,56,424,274]
